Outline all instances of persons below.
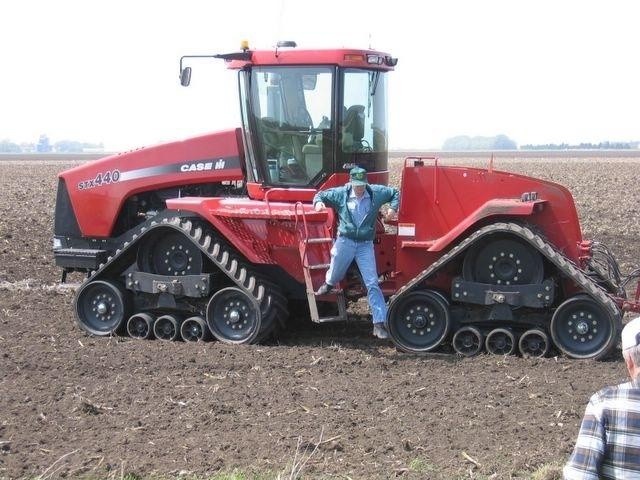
[564,317,640,480]
[312,167,401,341]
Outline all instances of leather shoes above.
[318,284,332,296]
[373,323,389,339]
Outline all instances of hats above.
[621,318,640,350]
[350,168,367,187]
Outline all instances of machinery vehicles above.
[51,39,640,361]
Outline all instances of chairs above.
[309,105,364,148]
